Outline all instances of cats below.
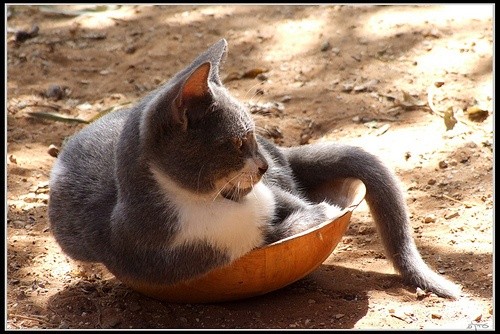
[47,38,462,302]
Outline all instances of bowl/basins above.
[101,174,368,302]
